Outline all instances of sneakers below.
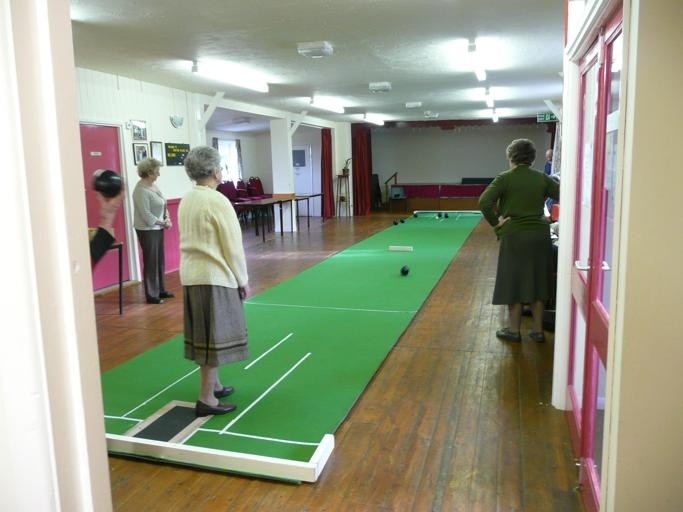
[497,327,544,343]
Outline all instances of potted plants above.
[340,156,353,175]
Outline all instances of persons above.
[543,148,553,173]
[177,145,251,418]
[478,138,560,343]
[89,187,126,272]
[131,157,174,305]
[521,172,560,319]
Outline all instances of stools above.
[335,174,350,218]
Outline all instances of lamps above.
[190,57,385,126]
[465,44,498,124]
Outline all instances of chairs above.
[216,176,273,225]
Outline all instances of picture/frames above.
[150,141,164,167]
[130,119,151,142]
[132,143,150,166]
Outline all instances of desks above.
[234,190,324,241]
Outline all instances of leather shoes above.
[196,399,235,416]
[147,292,173,303]
[214,387,232,398]
